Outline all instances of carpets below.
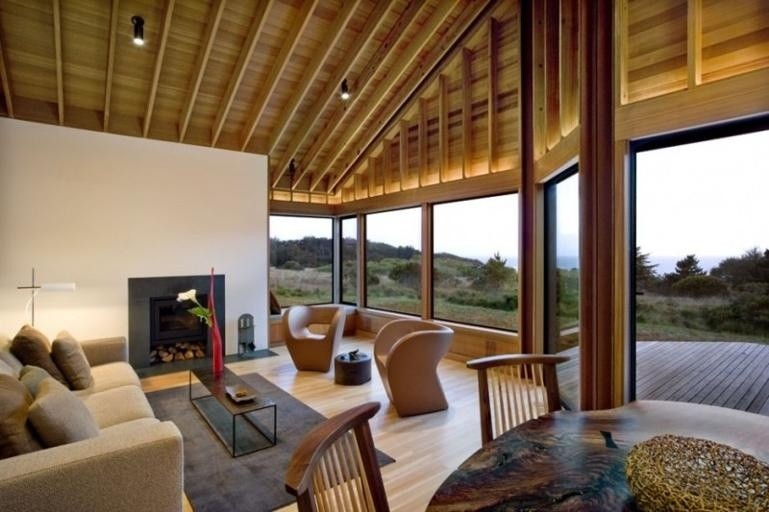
[141,369,398,511]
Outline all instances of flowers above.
[175,285,217,333]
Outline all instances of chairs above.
[278,399,393,511]
[280,303,347,373]
[369,315,457,417]
[461,352,573,446]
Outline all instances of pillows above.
[0,320,102,459]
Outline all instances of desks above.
[332,350,374,387]
[419,395,769,510]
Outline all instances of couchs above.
[0,327,190,512]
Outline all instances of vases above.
[206,266,227,383]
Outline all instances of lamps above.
[18,265,77,328]
[130,15,146,47]
[340,79,350,101]
